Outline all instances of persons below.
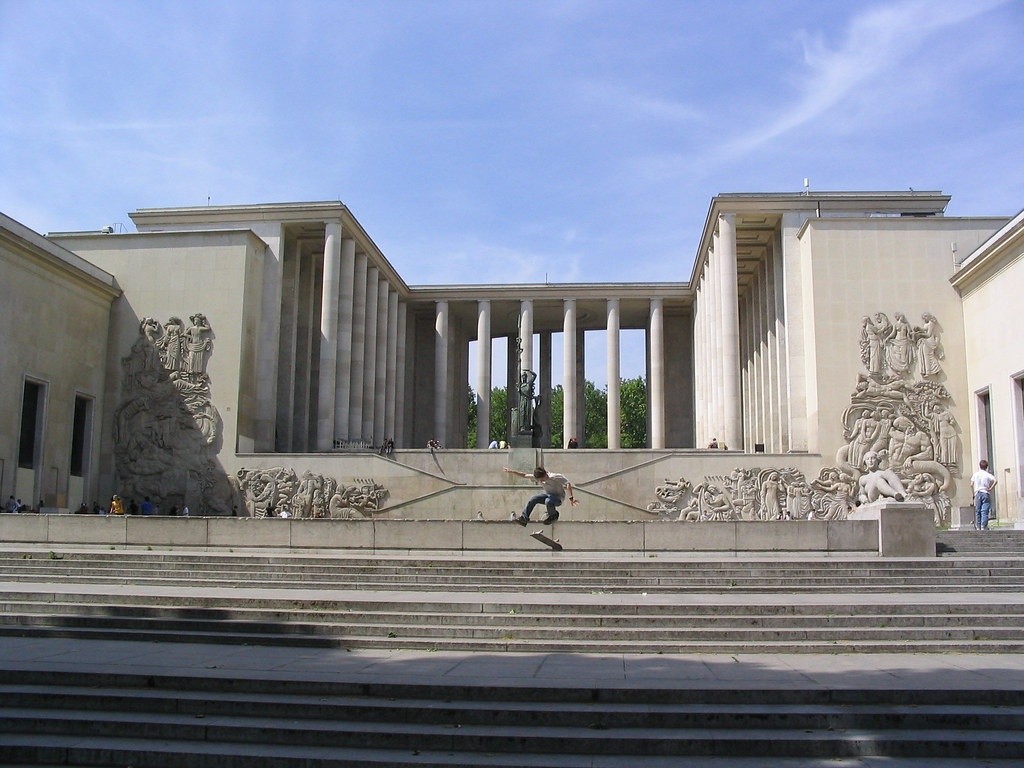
[2,494,283,518]
[427,438,578,449]
[514,368,538,433]
[112,314,388,522]
[386,438,394,453]
[504,466,580,527]
[647,312,961,530]
[970,460,997,531]
[708,438,718,448]
[378,439,388,454]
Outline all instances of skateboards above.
[530,529,563,551]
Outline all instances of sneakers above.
[515,517,529,528]
[544,512,559,525]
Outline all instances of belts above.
[978,491,983,493]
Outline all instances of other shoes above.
[977,527,988,530]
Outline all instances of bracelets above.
[568,497,574,500]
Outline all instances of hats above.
[113,495,118,500]
